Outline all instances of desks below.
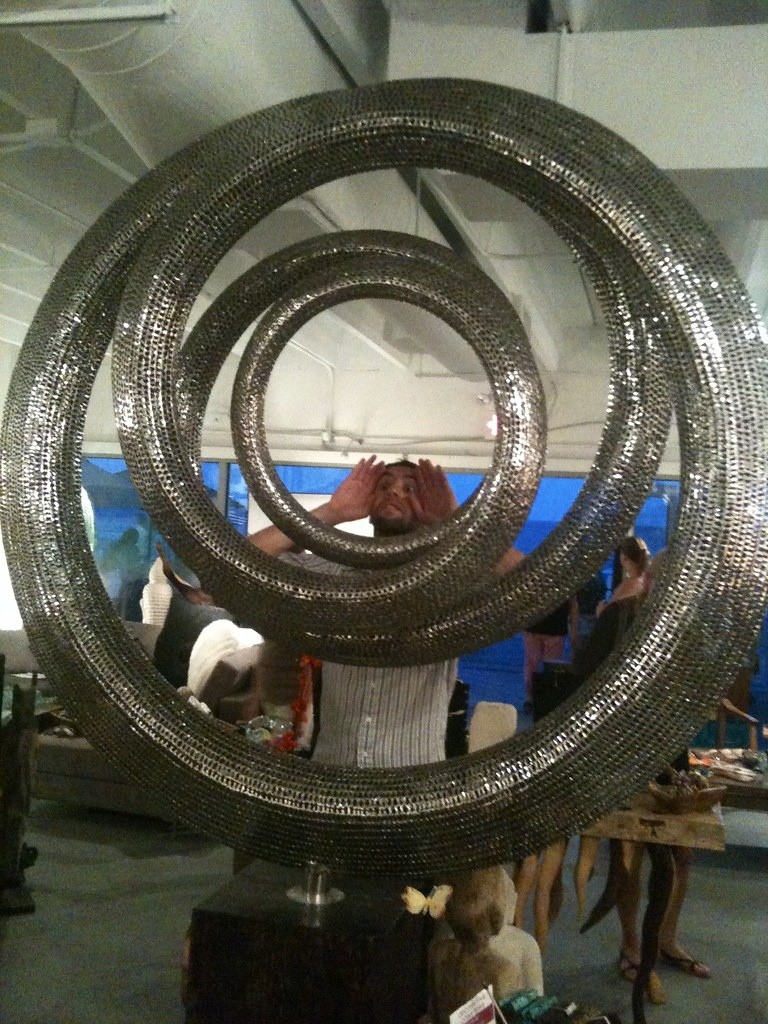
[578,784,728,1023]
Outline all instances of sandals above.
[660,950,710,977]
[617,948,642,982]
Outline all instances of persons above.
[521,592,578,715]
[612,747,710,984]
[595,535,653,620]
[236,454,529,772]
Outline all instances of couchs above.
[28,643,264,818]
